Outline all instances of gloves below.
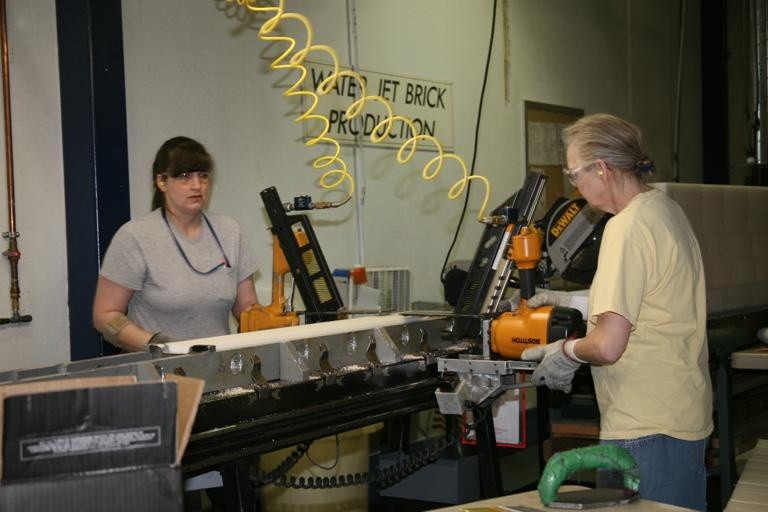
[520,338,589,394]
[507,284,588,325]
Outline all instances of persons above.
[92,136,260,353]
[520,113,715,512]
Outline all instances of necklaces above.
[161,206,231,274]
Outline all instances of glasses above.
[156,170,212,184]
[560,160,600,190]
